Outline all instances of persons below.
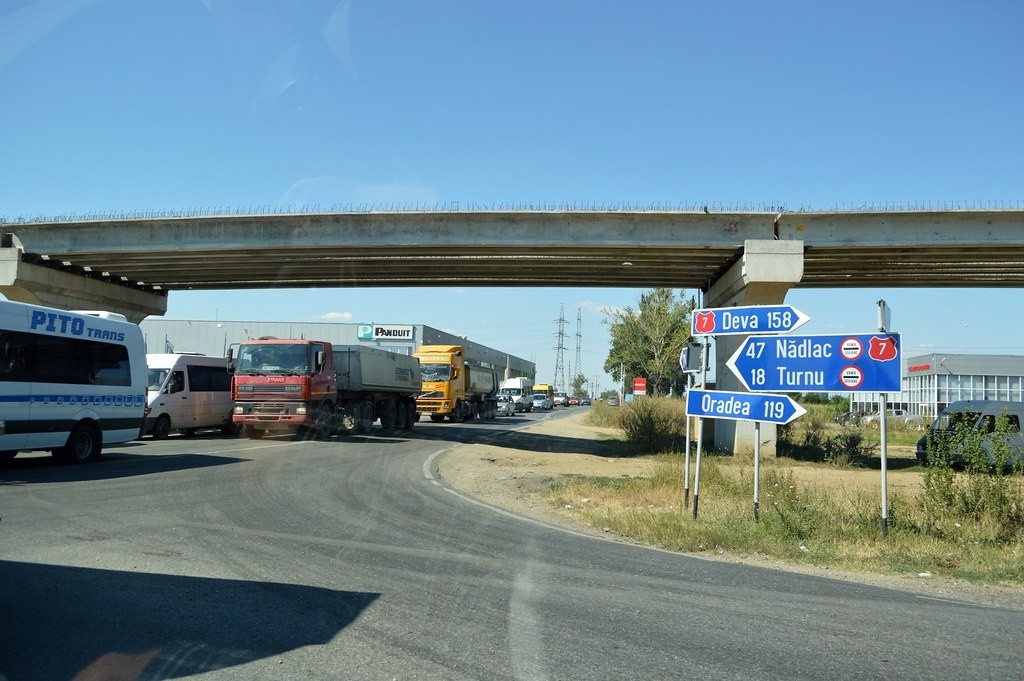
[438,368,450,382]
[294,356,309,374]
[161,370,180,393]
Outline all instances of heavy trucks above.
[223,335,424,441]
[410,343,499,422]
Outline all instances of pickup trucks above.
[860,408,922,429]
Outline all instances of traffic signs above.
[691,304,811,336]
[724,334,904,392]
[686,388,808,424]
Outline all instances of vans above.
[607,396,619,407]
[914,400,1024,476]
[0,300,149,460]
[531,383,556,409]
[144,352,251,436]
[498,376,535,411]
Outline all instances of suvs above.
[552,391,569,408]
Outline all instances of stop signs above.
[633,377,647,396]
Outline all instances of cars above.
[832,410,878,429]
[568,396,580,407]
[533,393,551,411]
[494,394,515,418]
[579,397,592,407]
